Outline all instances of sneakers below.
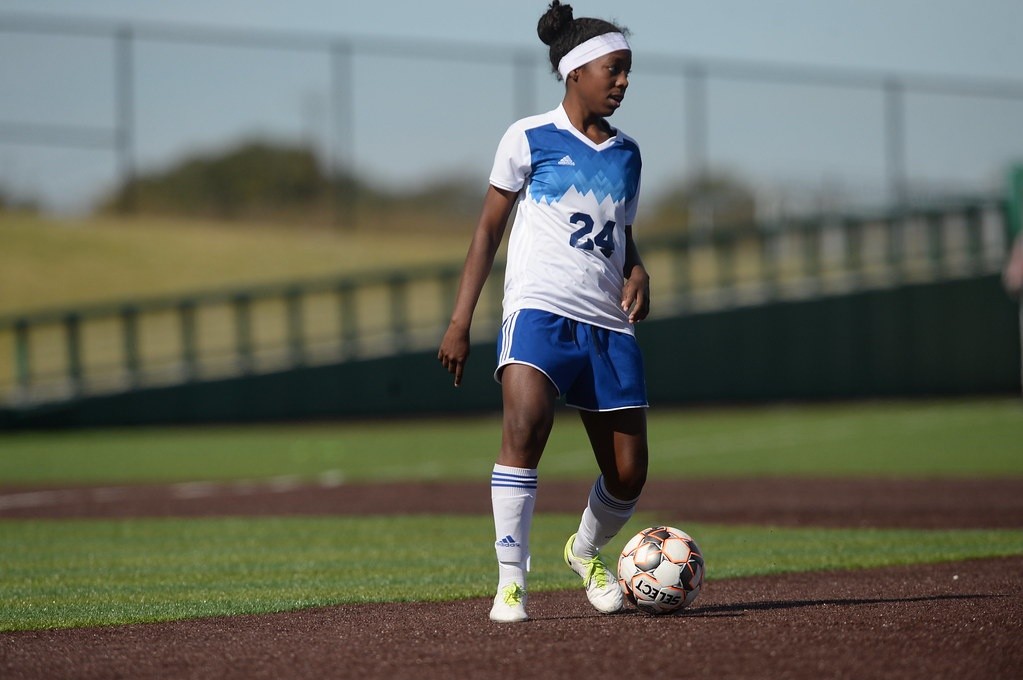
[564,533,623,614]
[490,582,529,623]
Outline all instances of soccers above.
[616,525,704,613]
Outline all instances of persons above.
[436,0,653,623]
